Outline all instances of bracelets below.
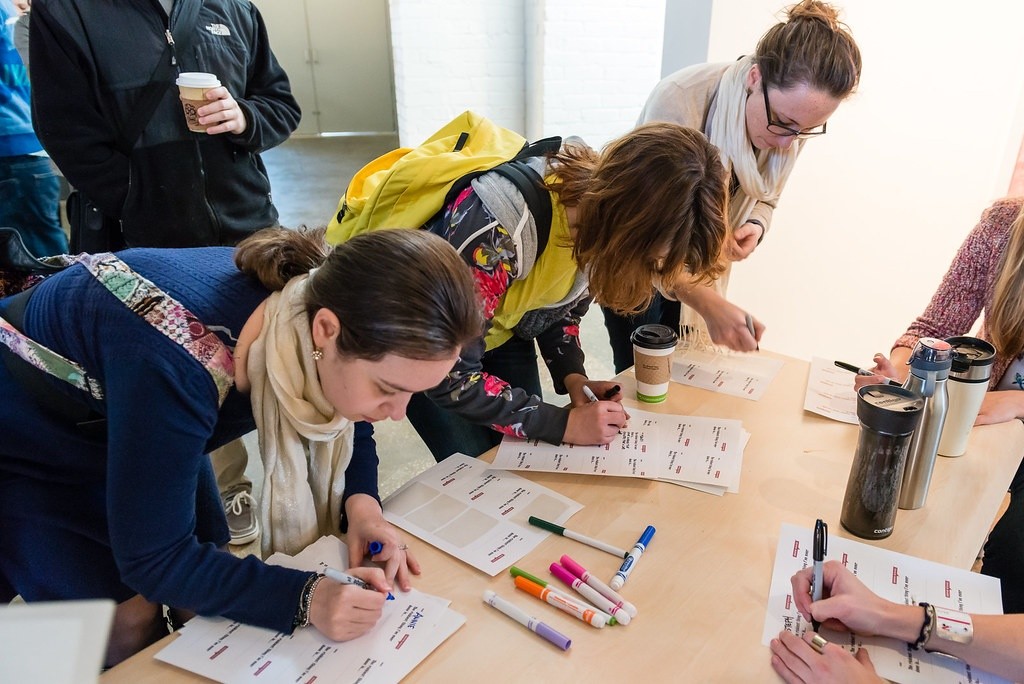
[905,602,934,651]
[925,605,974,661]
[296,573,328,627]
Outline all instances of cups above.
[630,323,679,403]
[176,71,223,132]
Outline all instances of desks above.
[97,330,1024,684]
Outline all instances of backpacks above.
[327,111,563,261]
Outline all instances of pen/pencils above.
[835,361,903,387]
[745,314,759,350]
[811,519,827,632]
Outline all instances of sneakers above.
[224,490,258,545]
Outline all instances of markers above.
[483,590,571,651]
[583,385,622,434]
[550,563,631,625]
[560,554,636,618]
[510,566,616,626]
[610,526,656,590]
[514,576,605,628]
[324,568,396,600]
[529,516,628,558]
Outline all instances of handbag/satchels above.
[0,228,234,411]
[66,187,128,255]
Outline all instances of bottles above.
[839,382,924,541]
[935,334,997,457]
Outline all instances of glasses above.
[762,85,826,139]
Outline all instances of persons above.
[0,0,304,547]
[402,122,726,466]
[600,0,863,379]
[771,560,1024,684]
[855,195,1024,428]
[2,224,485,680]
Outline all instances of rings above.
[809,635,829,650]
[399,543,408,551]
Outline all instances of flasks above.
[891,336,953,509]
